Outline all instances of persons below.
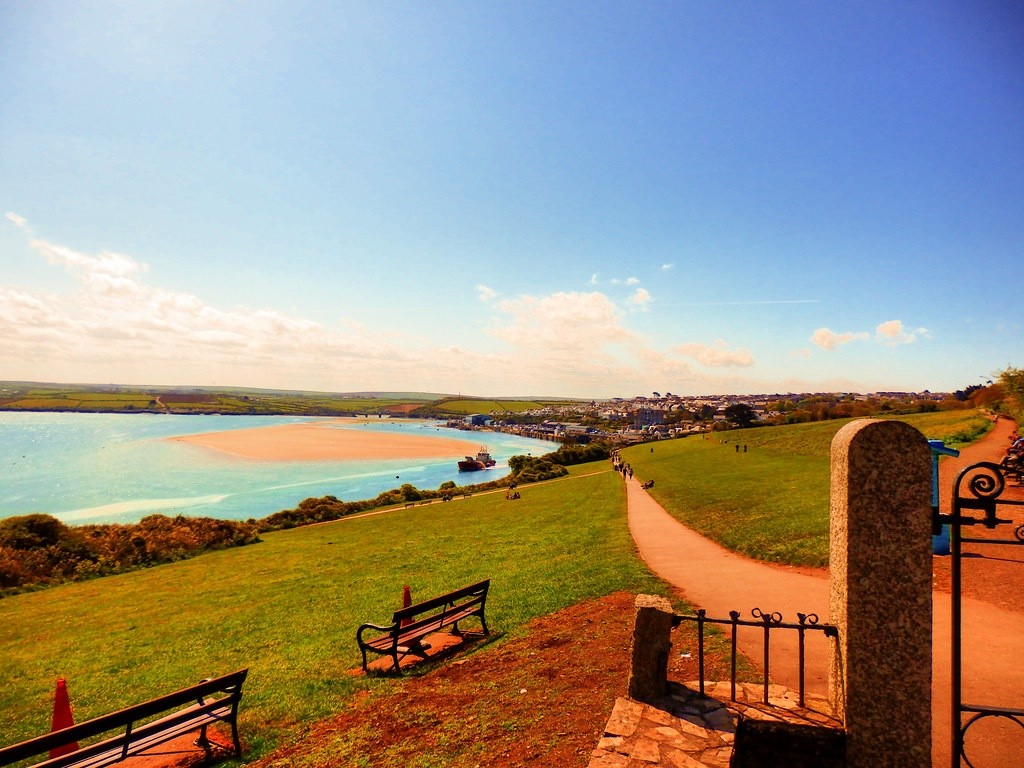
[641,477,654,489]
[744,445,747,452]
[724,440,727,444]
[505,492,520,500]
[736,443,739,452]
[611,449,632,479]
[997,431,1024,478]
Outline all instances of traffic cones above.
[391,585,432,654]
[45,676,81,762]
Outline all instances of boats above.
[475,445,496,468]
[457,456,486,472]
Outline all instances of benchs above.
[356,578,491,676]
[0,662,249,768]
